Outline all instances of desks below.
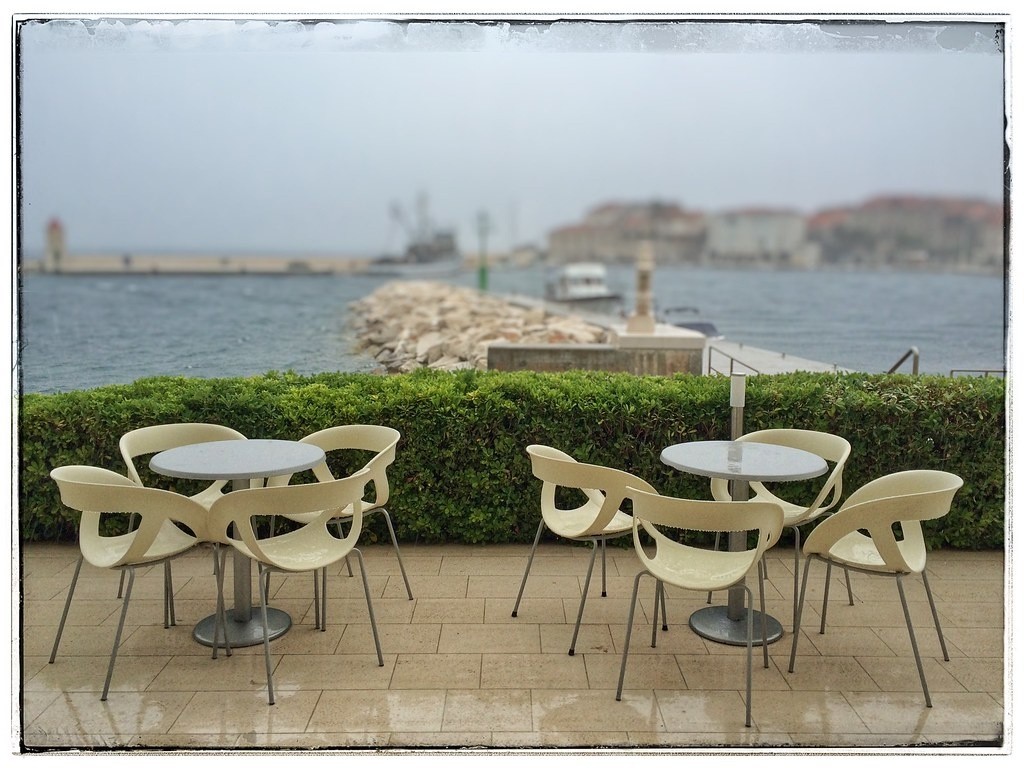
[661,441,827,646]
[150,438,326,649]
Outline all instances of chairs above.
[616,485,785,727]
[512,444,668,656]
[205,468,383,705]
[264,424,414,628]
[707,428,852,634]
[788,470,963,708]
[116,423,267,626]
[50,464,232,702]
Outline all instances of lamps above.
[729,373,746,407]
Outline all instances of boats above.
[544,262,625,307]
